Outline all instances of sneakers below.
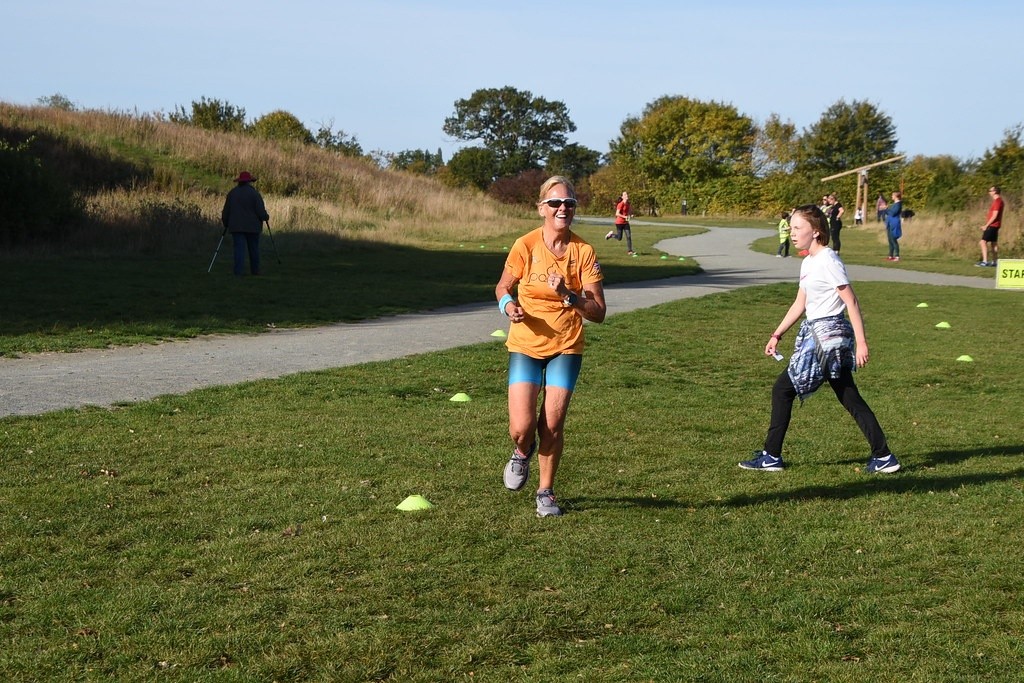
[536,489,562,518]
[990,263,996,267]
[863,454,900,474]
[503,440,537,491]
[974,262,988,267]
[738,450,784,471]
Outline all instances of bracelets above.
[771,334,781,340]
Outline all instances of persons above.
[776,208,796,258]
[738,204,902,473]
[884,191,903,261]
[605,191,635,255]
[974,186,1004,267]
[853,206,863,225]
[817,194,844,256]
[877,193,886,223]
[221,171,270,276]
[495,176,606,517]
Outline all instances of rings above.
[552,277,555,282]
[551,283,554,286]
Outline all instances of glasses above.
[540,198,578,208]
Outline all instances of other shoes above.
[605,231,614,240]
[785,255,792,258]
[628,251,635,255]
[777,254,783,257]
[883,257,900,262]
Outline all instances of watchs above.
[563,291,578,307]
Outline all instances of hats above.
[233,172,257,182]
